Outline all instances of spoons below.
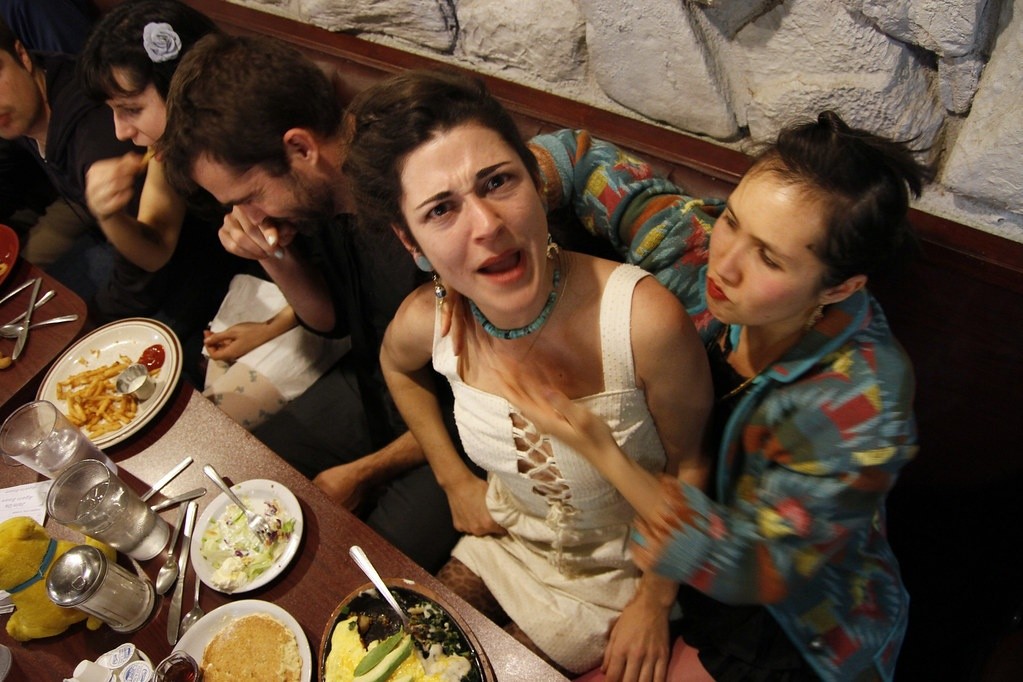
[156,500,189,595]
[179,574,205,641]
[0,313,79,338]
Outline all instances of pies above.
[197,614,303,682]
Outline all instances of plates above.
[163,599,311,682]
[0,224,19,287]
[33,317,183,450]
[190,479,302,593]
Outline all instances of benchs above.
[192,0,1022,682]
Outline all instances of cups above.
[62,643,152,682]
[153,650,202,682]
[45,459,170,561]
[115,363,157,402]
[0,400,118,476]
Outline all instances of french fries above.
[56,360,161,440]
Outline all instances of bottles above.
[45,545,156,634]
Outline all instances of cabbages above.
[202,497,296,591]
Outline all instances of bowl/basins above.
[318,578,496,682]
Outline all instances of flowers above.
[142,21,182,63]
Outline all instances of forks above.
[202,463,275,543]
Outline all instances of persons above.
[437,108,935,682]
[341,74,714,681]
[1,1,349,433]
[146,29,485,576]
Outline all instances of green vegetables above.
[391,591,482,682]
[339,594,403,645]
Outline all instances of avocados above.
[353,629,412,682]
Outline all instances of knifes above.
[12,277,42,361]
[167,500,197,645]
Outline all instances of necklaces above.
[725,349,769,398]
[487,275,567,362]
[467,260,562,338]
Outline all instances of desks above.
[0,381,573,682]
[0,258,89,403]
